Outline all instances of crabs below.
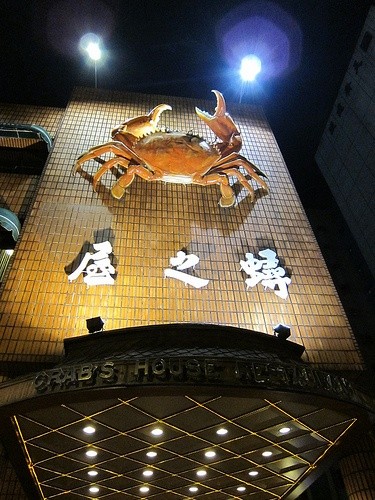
[72,89,272,208]
